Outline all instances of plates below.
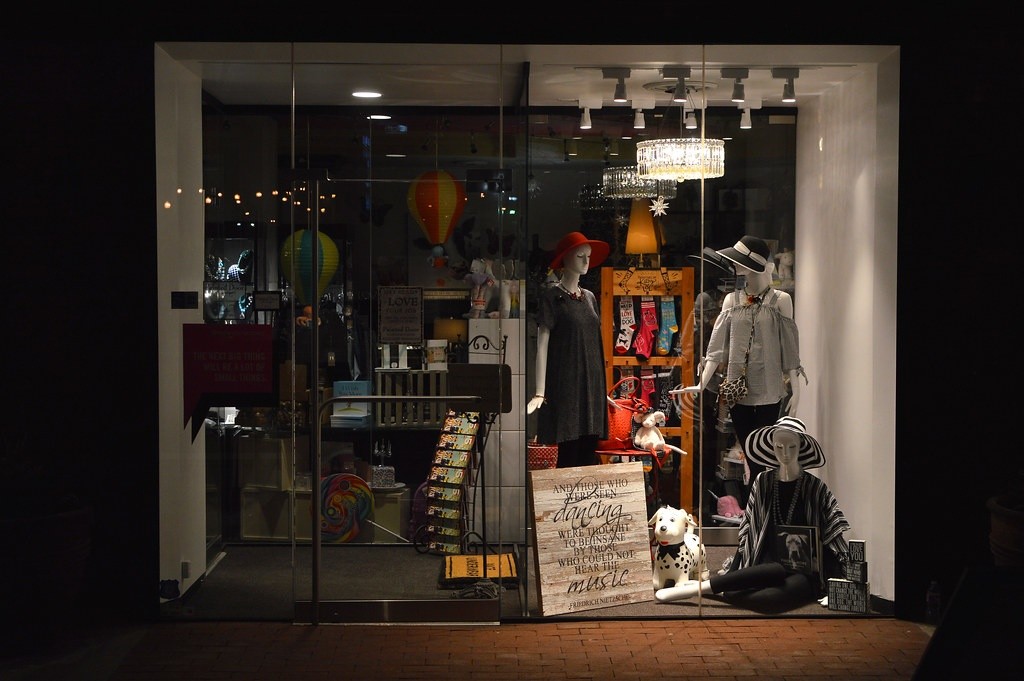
[365,481,405,491]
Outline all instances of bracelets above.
[534,395,547,405]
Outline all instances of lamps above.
[578,66,800,130]
[603,167,676,198]
[638,82,724,180]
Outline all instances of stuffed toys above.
[633,409,688,458]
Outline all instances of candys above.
[321,473,374,543]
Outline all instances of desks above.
[597,450,671,504]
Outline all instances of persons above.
[672,235,806,511]
[524,233,608,468]
[654,415,850,616]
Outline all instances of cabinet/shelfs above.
[602,268,694,513]
[412,333,507,553]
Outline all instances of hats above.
[550,232,610,268]
[717,235,769,275]
[746,417,826,470]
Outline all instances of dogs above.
[648,504,710,590]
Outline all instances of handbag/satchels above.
[720,376,749,406]
[607,377,649,441]
